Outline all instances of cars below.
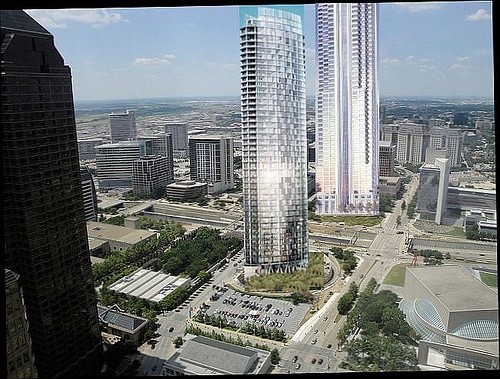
[149,291,333,372]
